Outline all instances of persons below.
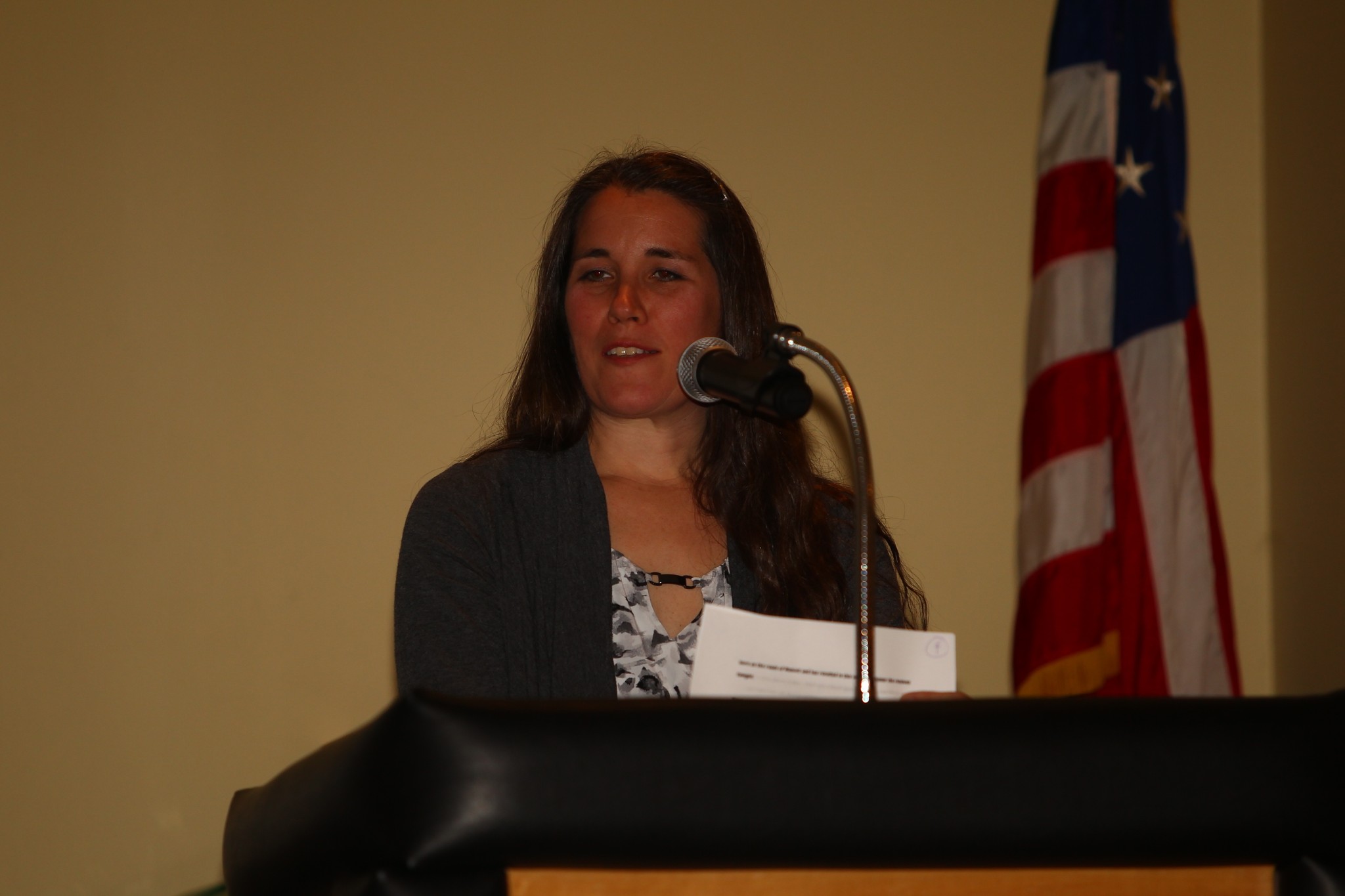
[389,148,935,706]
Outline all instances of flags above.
[1010,0,1250,702]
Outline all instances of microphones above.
[677,338,813,423]
